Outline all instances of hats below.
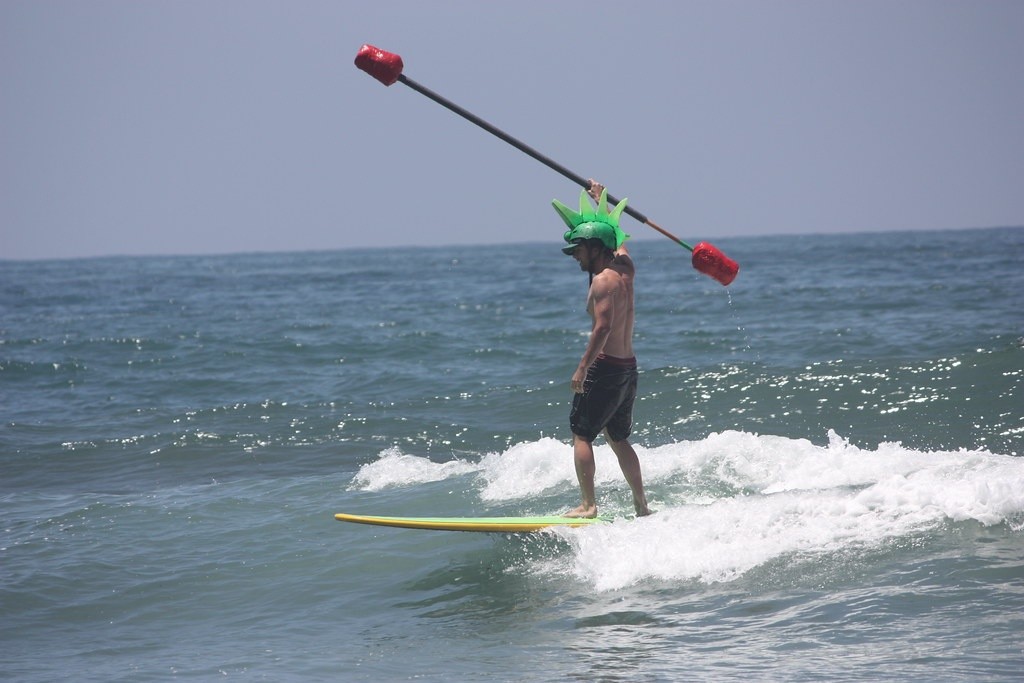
[552,188,630,251]
[562,238,582,256]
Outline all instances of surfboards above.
[334,513,617,532]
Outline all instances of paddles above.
[354,42,741,288]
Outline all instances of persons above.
[552,178,651,519]
